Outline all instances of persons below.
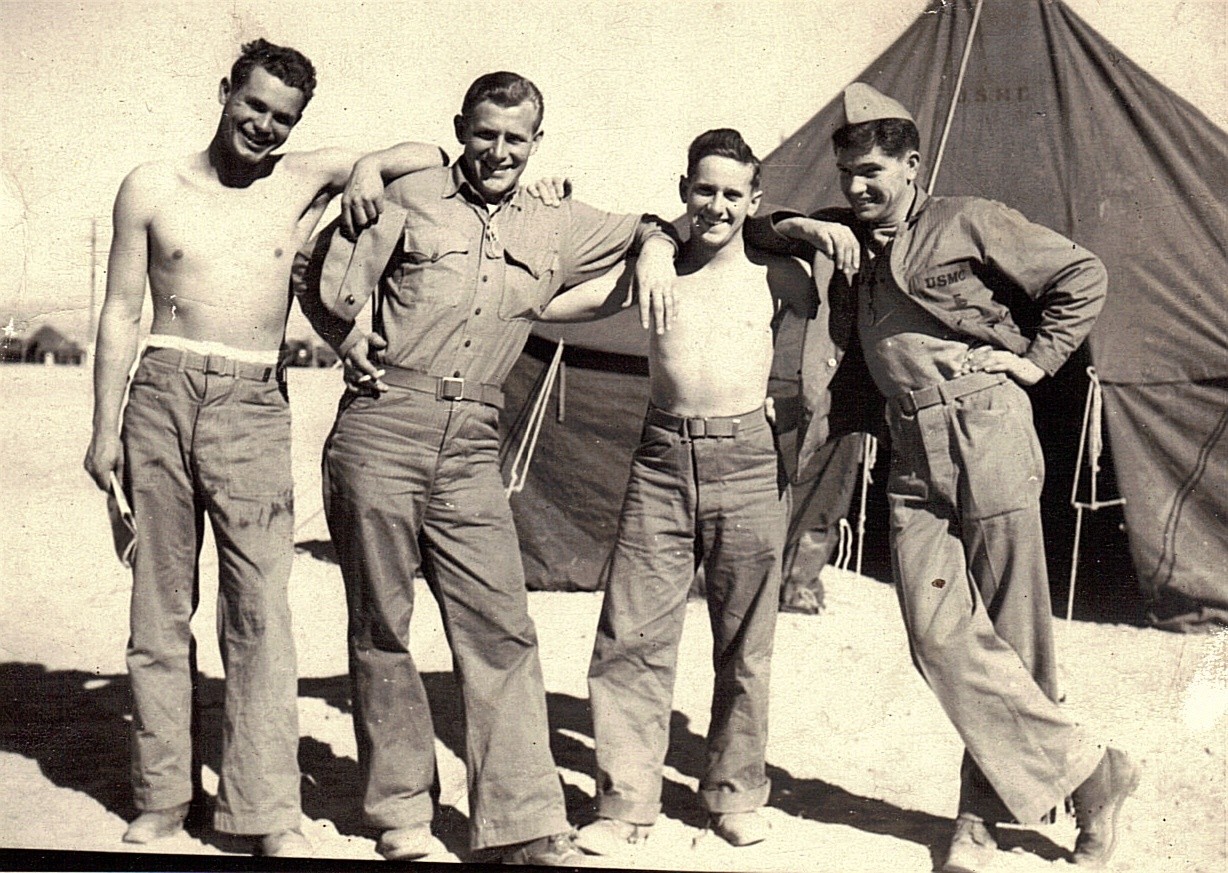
[291,70,680,867]
[83,39,449,857]
[523,128,815,857]
[745,82,1138,873]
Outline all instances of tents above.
[419,0,1228,631]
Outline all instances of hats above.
[830,81,918,139]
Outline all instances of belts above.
[886,371,1007,414]
[145,347,286,381]
[646,406,767,442]
[369,362,506,408]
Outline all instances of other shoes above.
[1065,747,1141,869]
[377,820,433,858]
[573,816,652,855]
[711,810,773,846]
[502,833,601,867]
[231,831,314,856]
[940,814,1000,873]
[122,801,189,843]
